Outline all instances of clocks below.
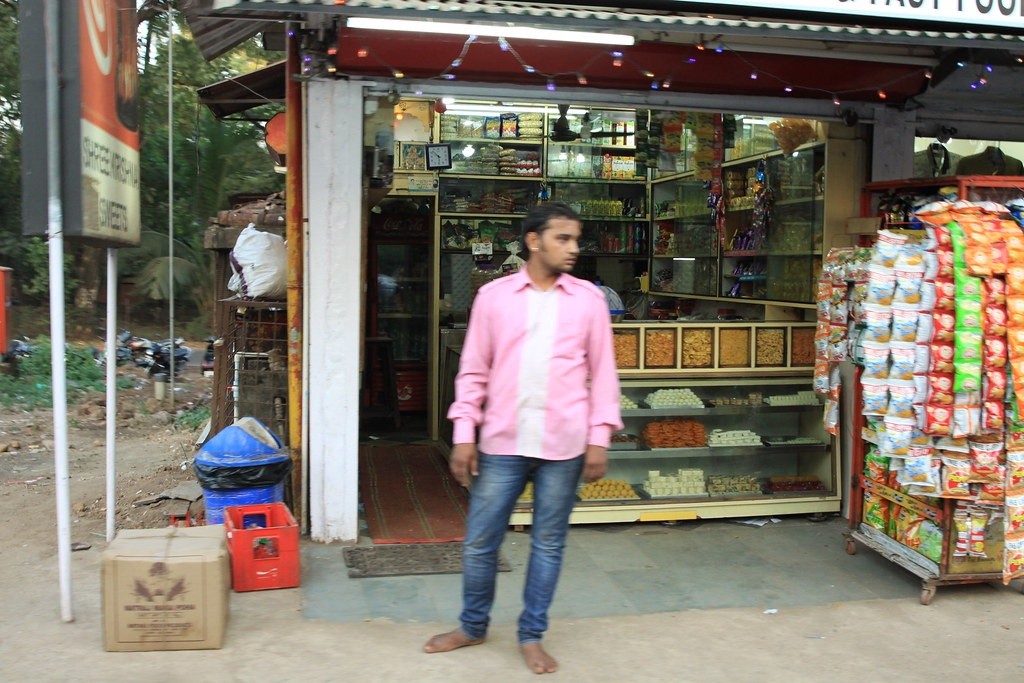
[425,144,452,170]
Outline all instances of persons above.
[425,201,625,675]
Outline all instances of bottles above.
[570,197,637,216]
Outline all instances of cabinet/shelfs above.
[427,101,865,441]
[507,318,843,531]
[844,174,1024,604]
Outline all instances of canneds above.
[607,223,642,254]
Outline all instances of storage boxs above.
[224,501,301,592]
[99,524,231,653]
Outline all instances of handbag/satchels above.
[229,222,288,303]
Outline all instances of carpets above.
[342,542,512,578]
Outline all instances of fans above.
[496,106,634,141]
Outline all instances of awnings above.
[229,0,1024,67]
[195,60,287,120]
[186,5,291,62]
